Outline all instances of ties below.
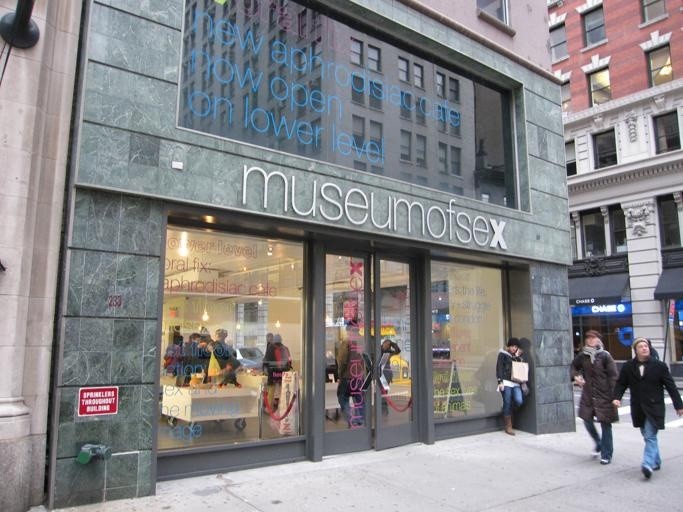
[640,365,645,378]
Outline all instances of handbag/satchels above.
[511,362,529,382]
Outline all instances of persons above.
[162,327,236,386]
[337,319,365,429]
[569,329,619,464]
[263,332,292,414]
[496,337,530,436]
[381,339,401,416]
[648,339,659,359]
[610,337,683,479]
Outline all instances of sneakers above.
[591,447,609,464]
[640,464,660,478]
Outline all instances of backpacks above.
[274,347,291,370]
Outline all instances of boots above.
[503,416,515,436]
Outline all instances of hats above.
[214,329,227,336]
[633,338,648,346]
[586,330,601,339]
[508,338,520,346]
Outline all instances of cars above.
[237,347,265,368]
